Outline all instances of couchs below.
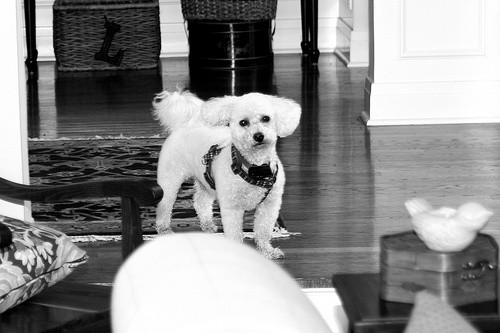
[109,231,330,333]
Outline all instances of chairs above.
[0,173,164,333]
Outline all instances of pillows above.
[404,287,480,332]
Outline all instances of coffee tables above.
[331,267,500,332]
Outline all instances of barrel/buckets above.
[184,16,276,72]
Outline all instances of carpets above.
[25,129,293,241]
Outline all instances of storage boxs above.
[376,230,500,310]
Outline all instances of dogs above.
[150,83,302,262]
